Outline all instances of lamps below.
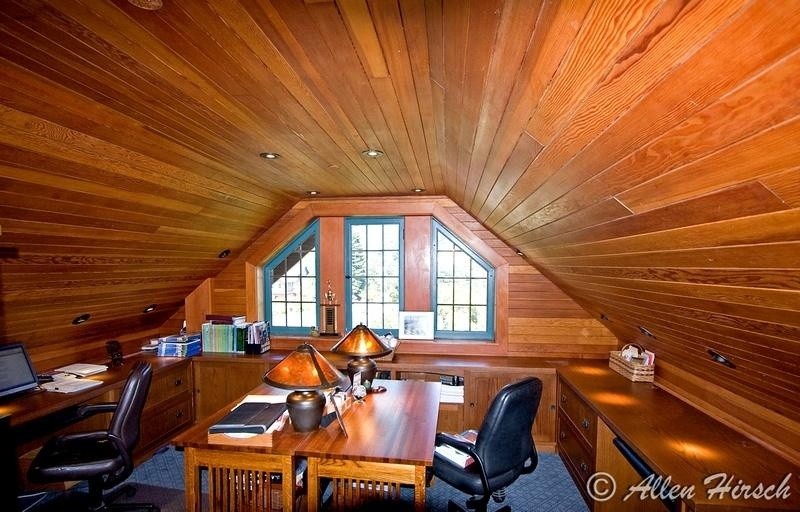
[331,321,394,387]
[261,342,345,428]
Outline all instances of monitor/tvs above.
[0,342,40,398]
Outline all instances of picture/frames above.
[398,311,435,340]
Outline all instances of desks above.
[540,356,800,511]
[1,342,192,512]
[169,375,352,511]
[294,378,443,511]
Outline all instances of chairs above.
[433,374,546,511]
[29,359,160,510]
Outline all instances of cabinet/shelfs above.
[462,366,555,452]
[329,365,463,438]
[194,360,267,422]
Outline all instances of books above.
[141,313,273,358]
[435,428,479,470]
[421,373,465,405]
[630,349,656,365]
